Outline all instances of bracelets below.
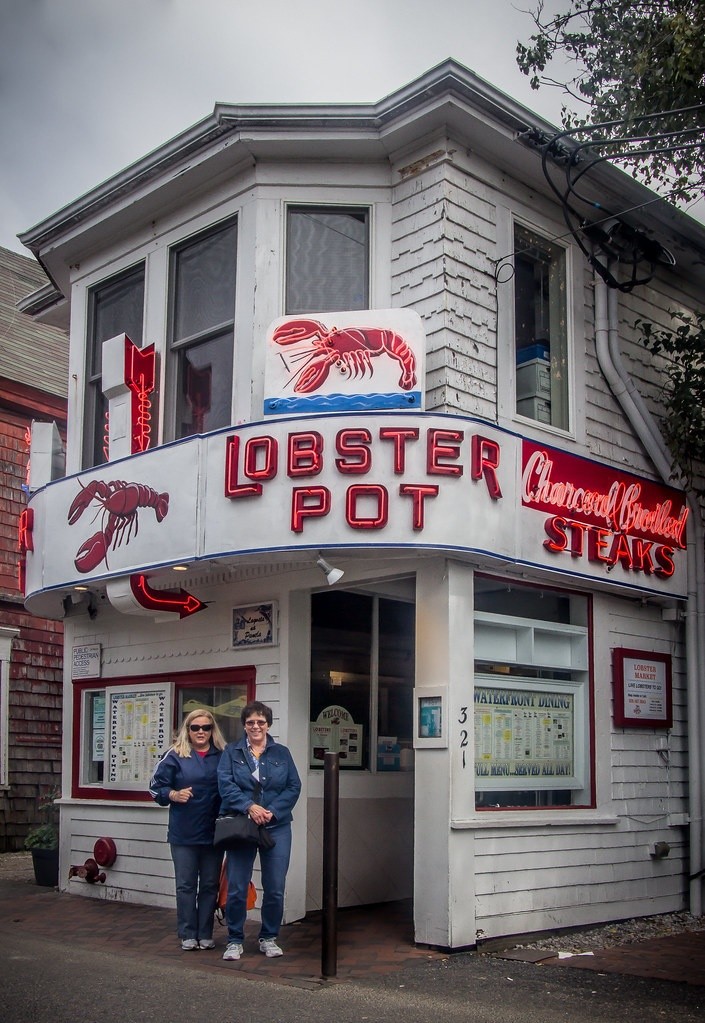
[170,791,176,800]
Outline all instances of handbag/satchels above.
[215,854,257,911]
[214,814,262,848]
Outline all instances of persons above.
[149,709,228,950]
[217,701,302,960]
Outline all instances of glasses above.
[245,721,267,726]
[189,725,213,731]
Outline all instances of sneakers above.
[181,939,199,950]
[199,939,215,949]
[258,937,283,958]
[223,943,243,960]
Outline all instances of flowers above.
[25,782,61,849]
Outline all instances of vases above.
[28,846,59,887]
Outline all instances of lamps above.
[316,552,345,586]
